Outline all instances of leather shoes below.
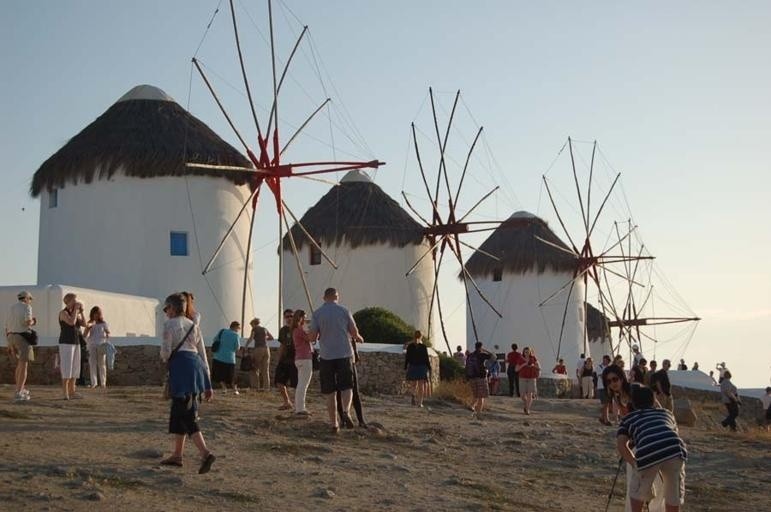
[331,425,340,432]
[343,412,354,429]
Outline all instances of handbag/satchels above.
[241,348,252,372]
[211,329,224,351]
[168,323,195,362]
[23,325,39,345]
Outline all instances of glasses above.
[285,314,293,318]
[605,376,620,385]
[163,305,171,313]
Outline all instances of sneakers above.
[64,395,69,400]
[14,393,30,401]
[278,403,293,411]
[160,457,184,467]
[22,389,29,394]
[70,392,82,399]
[296,410,312,418]
[198,452,216,474]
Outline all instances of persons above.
[454,345,464,363]
[618,361,624,369]
[515,347,538,415]
[181,291,213,401]
[481,347,497,410]
[763,387,771,429]
[290,310,319,415]
[310,288,364,432]
[83,306,110,388]
[648,360,657,384]
[766,403,771,432]
[507,343,522,397]
[310,331,321,370]
[721,371,741,432]
[678,359,687,371]
[275,309,298,410]
[212,321,241,395]
[245,348,260,391]
[466,341,491,415]
[490,359,501,396]
[58,293,87,400]
[591,359,598,397]
[709,371,717,386]
[230,346,244,389]
[616,387,686,512]
[692,362,699,370]
[576,353,588,399]
[582,357,594,399]
[159,292,216,474]
[464,350,469,366]
[602,364,666,512]
[337,330,369,428]
[651,359,673,416]
[632,345,644,371]
[630,358,648,384]
[608,352,622,414]
[417,339,431,399]
[4,290,37,400]
[716,362,729,384]
[403,330,433,408]
[597,355,612,426]
[531,349,541,400]
[551,359,567,397]
[245,317,274,392]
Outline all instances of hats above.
[632,344,639,350]
[18,290,35,300]
